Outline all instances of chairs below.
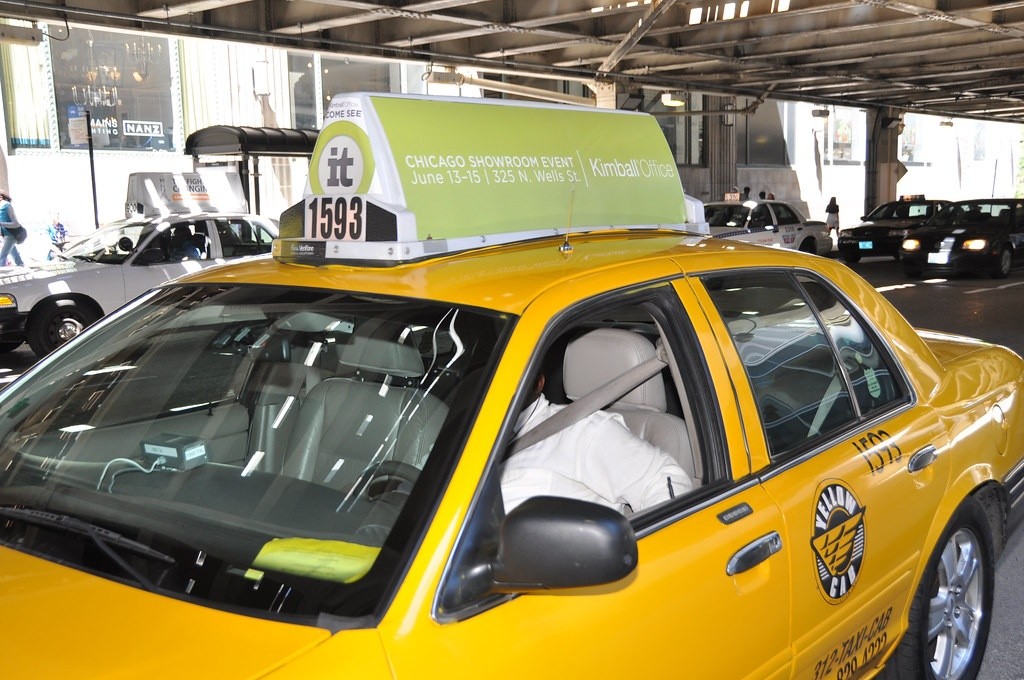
[563,328,695,473]
[281,318,452,489]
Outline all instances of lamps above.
[660,93,685,107]
[72,34,118,107]
[254,61,271,95]
[882,118,902,129]
[125,39,163,83]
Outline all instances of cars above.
[837,200,965,264]
[0,174,279,359]
[702,200,834,256]
[0,92,1024,680]
[898,197,1024,278]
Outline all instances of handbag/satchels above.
[9,226,28,245]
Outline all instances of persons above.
[826,197,839,236]
[44,210,66,262]
[343,327,692,520]
[732,186,751,217]
[0,190,26,266]
[758,192,773,216]
